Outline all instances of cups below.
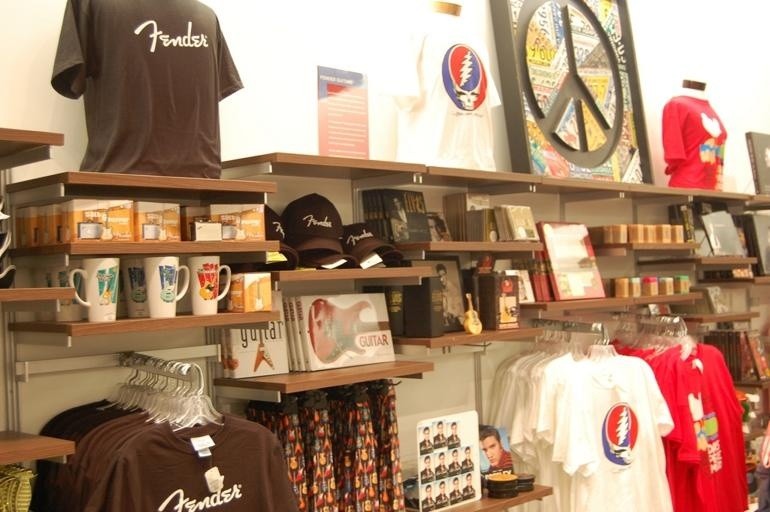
[45,254,232,319]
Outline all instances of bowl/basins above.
[487,471,535,497]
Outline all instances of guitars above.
[309,298,392,363]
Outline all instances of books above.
[363,188,540,243]
[746,132,770,195]
[668,201,770,383]
[514,221,604,300]
[218,293,395,378]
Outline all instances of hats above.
[299,249,405,271]
[254,203,299,271]
[279,193,345,255]
[340,222,396,261]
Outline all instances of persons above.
[435,263,463,324]
[479,429,514,474]
[419,420,475,512]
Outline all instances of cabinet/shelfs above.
[0,126,770,512]
